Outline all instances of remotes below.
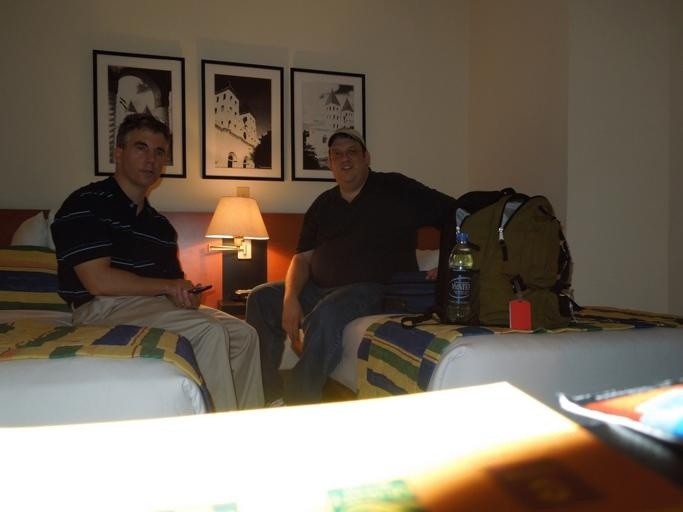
[188,285,212,294]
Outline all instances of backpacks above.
[460,192,560,328]
[400,188,580,330]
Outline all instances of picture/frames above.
[201,60,283,181]
[93,49,186,177]
[291,68,366,181]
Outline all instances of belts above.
[74,293,95,308]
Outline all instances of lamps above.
[204,197,270,260]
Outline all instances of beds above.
[0,311,214,430]
[328,305,683,409]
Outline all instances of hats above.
[328,128,366,148]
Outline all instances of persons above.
[49,112,265,411]
[243,127,451,401]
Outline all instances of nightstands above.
[217,299,246,315]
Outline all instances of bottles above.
[445,231,474,324]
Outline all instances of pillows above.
[0,245,73,314]
[46,208,58,251]
[10,211,48,246]
[416,248,440,272]
